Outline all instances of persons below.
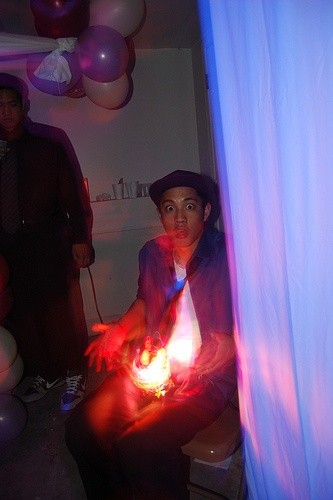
[65,170,239,500]
[0,72,96,411]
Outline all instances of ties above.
[0,143,20,236]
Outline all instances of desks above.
[76,196,165,335]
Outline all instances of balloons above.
[0,257,28,439]
[26,0,147,108]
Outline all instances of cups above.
[112,184,123,200]
[142,183,152,197]
[125,181,138,199]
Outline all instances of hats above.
[149,170,209,206]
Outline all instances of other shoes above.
[21,375,65,404]
[60,373,86,412]
